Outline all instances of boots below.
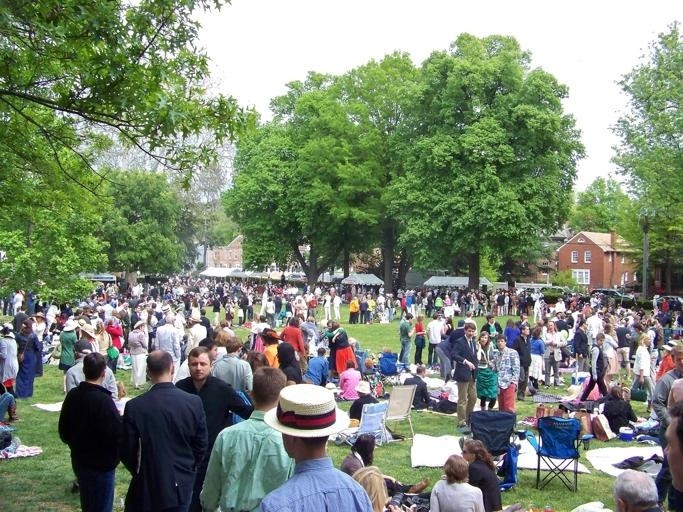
[8,402,23,422]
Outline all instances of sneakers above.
[457,425,471,434]
[544,386,558,390]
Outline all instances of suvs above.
[541,285,572,294]
[590,289,632,300]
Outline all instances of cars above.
[651,296,683,311]
[524,286,542,293]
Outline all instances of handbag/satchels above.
[107,346,119,360]
[18,353,24,362]
[51,344,62,358]
[593,409,616,442]
[631,382,647,401]
[537,404,593,435]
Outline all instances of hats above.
[326,382,343,392]
[162,305,171,313]
[263,383,351,437]
[0,322,15,338]
[188,316,202,323]
[355,381,370,393]
[662,340,679,352]
[62,319,96,339]
[31,312,45,319]
[134,321,145,329]
[258,328,281,340]
[75,340,92,354]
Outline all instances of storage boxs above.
[571,372,589,384]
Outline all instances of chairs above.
[334,400,389,448]
[386,381,419,441]
[380,357,406,385]
[527,415,593,494]
